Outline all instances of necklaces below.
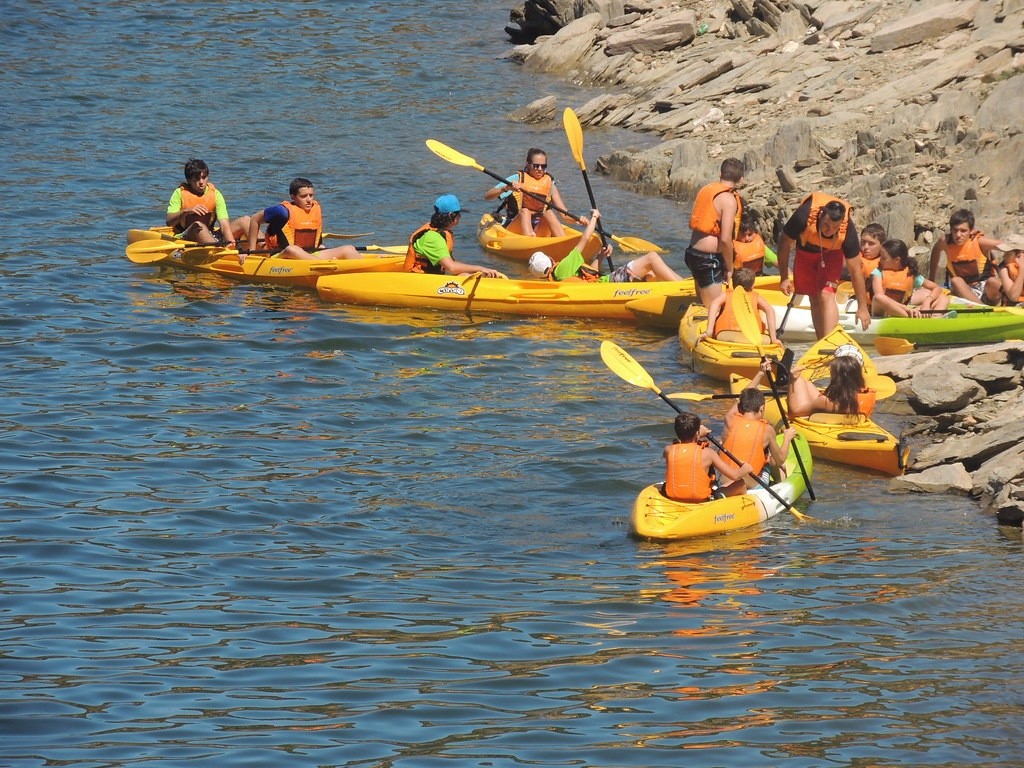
[818,223,836,268]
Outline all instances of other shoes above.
[943,311,958,319]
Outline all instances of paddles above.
[776,292,797,341]
[424,137,663,254]
[124,231,375,264]
[599,338,815,521]
[656,374,898,402]
[180,244,410,265]
[848,306,1024,317]
[561,106,617,273]
[873,335,1024,356]
[836,281,858,305]
[731,284,818,502]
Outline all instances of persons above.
[527,207,686,283]
[860,223,958,318]
[732,213,793,277]
[777,191,871,341]
[712,360,800,489]
[166,157,271,258]
[929,208,1004,307]
[485,147,590,237]
[995,234,1024,306]
[685,157,744,309]
[248,177,363,259]
[695,267,784,348]
[402,194,500,279]
[662,410,754,504]
[788,356,876,422]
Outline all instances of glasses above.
[530,162,547,169]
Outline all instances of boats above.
[621,273,1024,350]
[678,302,789,388]
[126,224,410,287]
[792,323,880,416]
[316,269,793,320]
[630,430,815,543]
[476,213,605,269]
[724,374,913,477]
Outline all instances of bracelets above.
[592,215,598,219]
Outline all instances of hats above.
[435,195,470,213]
[529,251,552,277]
[996,234,1024,251]
